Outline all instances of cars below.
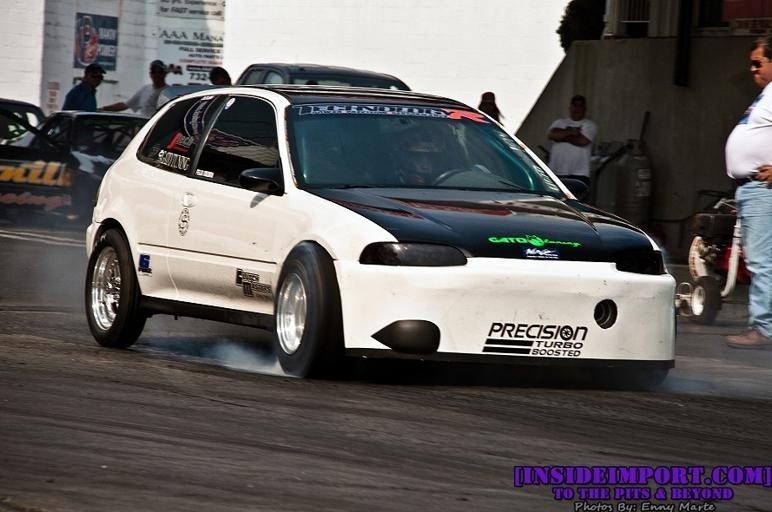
[0,63,676,386]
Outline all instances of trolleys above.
[676,195,749,322]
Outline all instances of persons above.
[97,59,171,117]
[724,31,771,348]
[61,63,108,146]
[472,91,504,172]
[545,94,596,202]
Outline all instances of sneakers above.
[726,327,772,351]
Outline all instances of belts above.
[736,173,761,187]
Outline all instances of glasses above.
[751,58,769,67]
[88,73,103,80]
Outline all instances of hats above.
[150,60,165,69]
[86,63,106,74]
[571,94,586,105]
